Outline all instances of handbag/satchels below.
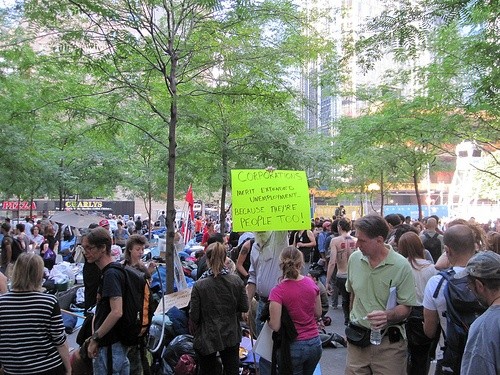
[173,355,196,375]
[163,334,196,367]
[76,313,94,346]
[252,319,273,362]
[345,323,372,348]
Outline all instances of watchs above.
[92,333,100,343]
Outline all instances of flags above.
[185,185,194,220]
[184,214,192,246]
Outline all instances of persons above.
[396,232,440,375]
[178,218,183,228]
[345,215,417,375]
[289,215,358,325]
[0,253,72,375]
[88,214,143,240]
[0,215,74,294]
[241,166,289,375]
[82,227,130,375]
[194,214,263,285]
[188,242,249,375]
[120,234,156,274]
[423,224,488,375]
[335,205,345,217]
[385,213,500,272]
[159,211,166,227]
[268,246,323,375]
[453,251,500,375]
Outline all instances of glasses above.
[79,245,98,254]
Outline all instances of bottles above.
[370,324,382,345]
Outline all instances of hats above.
[99,219,110,227]
[323,221,332,227]
[466,250,500,278]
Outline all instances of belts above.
[381,329,388,336]
[260,296,269,302]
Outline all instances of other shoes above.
[328,331,345,348]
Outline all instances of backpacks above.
[422,231,441,263]
[100,262,153,347]
[437,269,489,367]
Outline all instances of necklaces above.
[490,296,500,306]
